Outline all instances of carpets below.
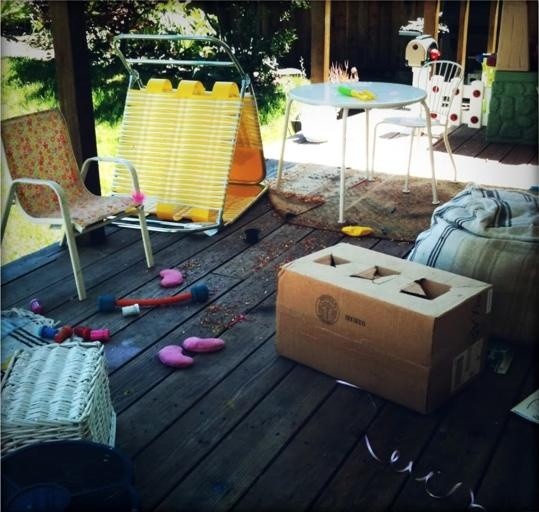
[265,163,465,244]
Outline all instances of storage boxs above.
[275,242,494,418]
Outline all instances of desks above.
[276,82,442,224]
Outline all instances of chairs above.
[370,62,468,201]
[0,113,154,299]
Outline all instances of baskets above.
[0,341,118,459]
